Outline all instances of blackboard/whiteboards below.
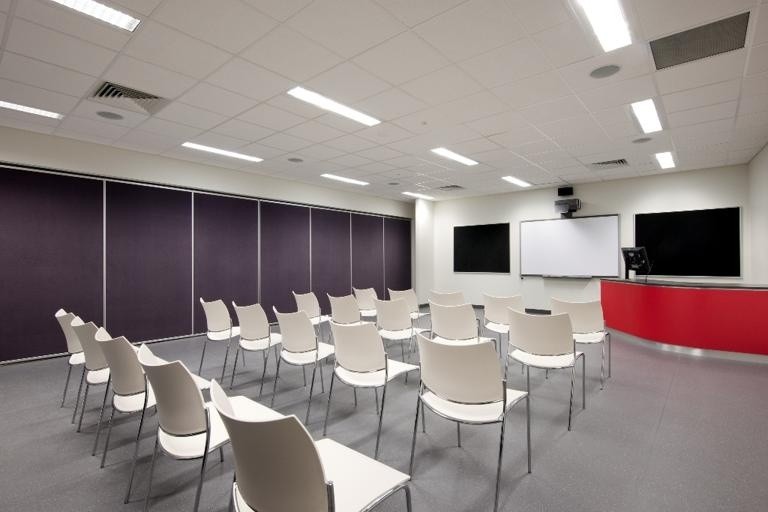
[519,212,621,278]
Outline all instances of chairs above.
[387,288,431,327]
[408,329,531,512]
[67,315,138,457]
[198,297,246,386]
[505,304,586,432]
[229,300,282,401]
[326,292,377,326]
[322,314,427,460]
[54,308,86,425]
[292,291,333,343]
[427,299,498,351]
[374,299,431,363]
[352,286,379,311]
[430,288,464,306]
[270,305,335,426]
[209,378,412,512]
[91,326,211,503]
[483,295,526,360]
[128,342,287,511]
[550,296,611,391]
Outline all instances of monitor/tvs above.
[622,246,650,276]
[632,204,743,278]
[451,222,511,274]
